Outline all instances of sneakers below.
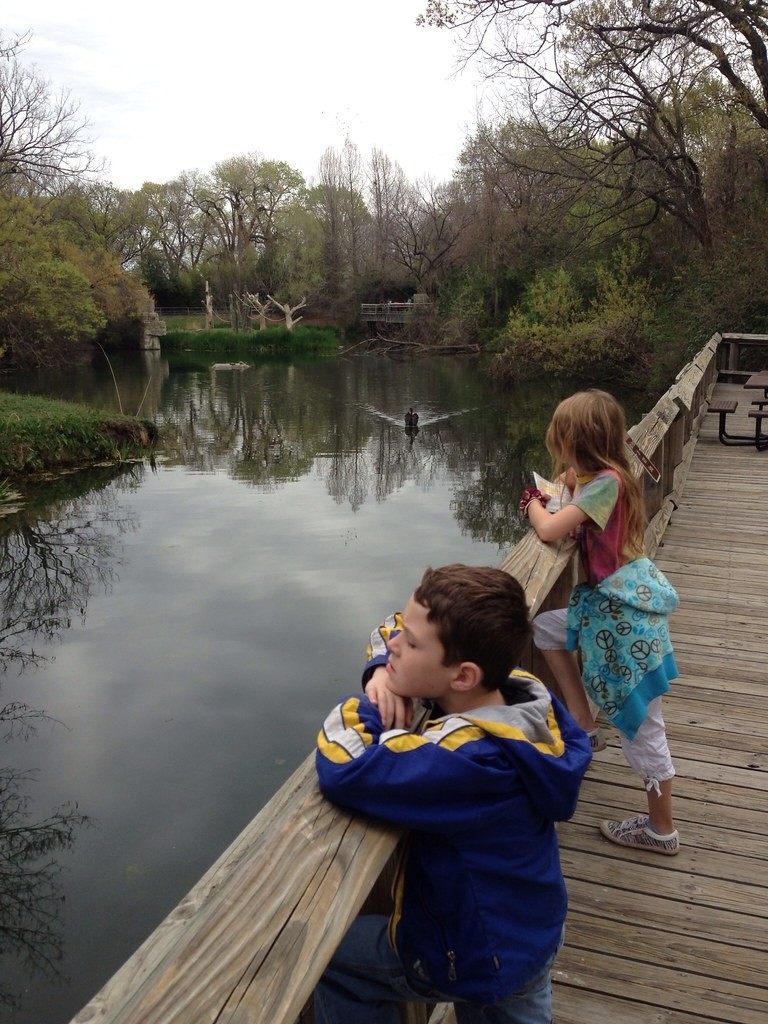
[601,816,679,855]
[585,726,604,754]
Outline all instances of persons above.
[528,388,681,855]
[311,564,592,1024]
[409,408,414,415]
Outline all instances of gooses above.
[404,408,419,426]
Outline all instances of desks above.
[744,375,768,451]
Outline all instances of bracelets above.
[520,488,546,518]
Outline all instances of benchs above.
[748,410,768,449]
[751,398,768,433]
[707,400,738,440]
